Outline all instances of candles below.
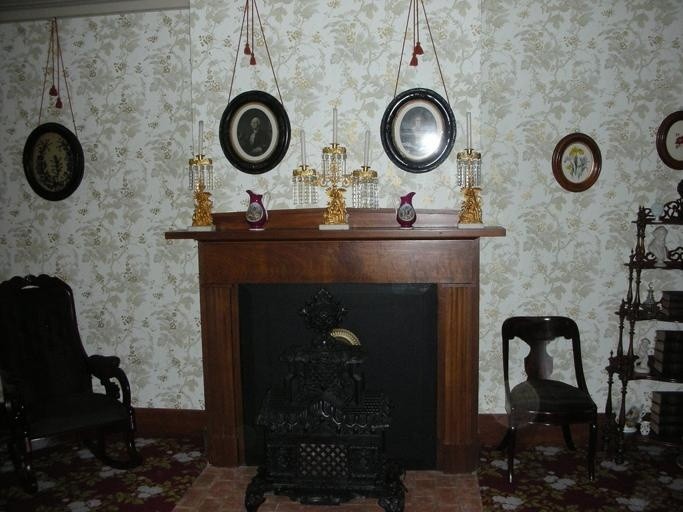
[333,107,338,144]
[362,130,369,165]
[299,130,305,165]
[196,120,203,156]
[466,113,472,151]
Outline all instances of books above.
[650,392,683,436]
[651,330,682,376]
[660,292,682,317]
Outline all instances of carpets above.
[171,460,483,512]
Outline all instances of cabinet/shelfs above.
[601,177,683,465]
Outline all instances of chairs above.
[0,272,138,493]
[493,317,598,484]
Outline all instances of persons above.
[633,337,650,374]
[649,226,667,268]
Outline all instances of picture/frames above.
[218,90,292,176]
[551,131,602,193]
[21,123,84,201]
[656,110,683,171]
[379,88,456,174]
